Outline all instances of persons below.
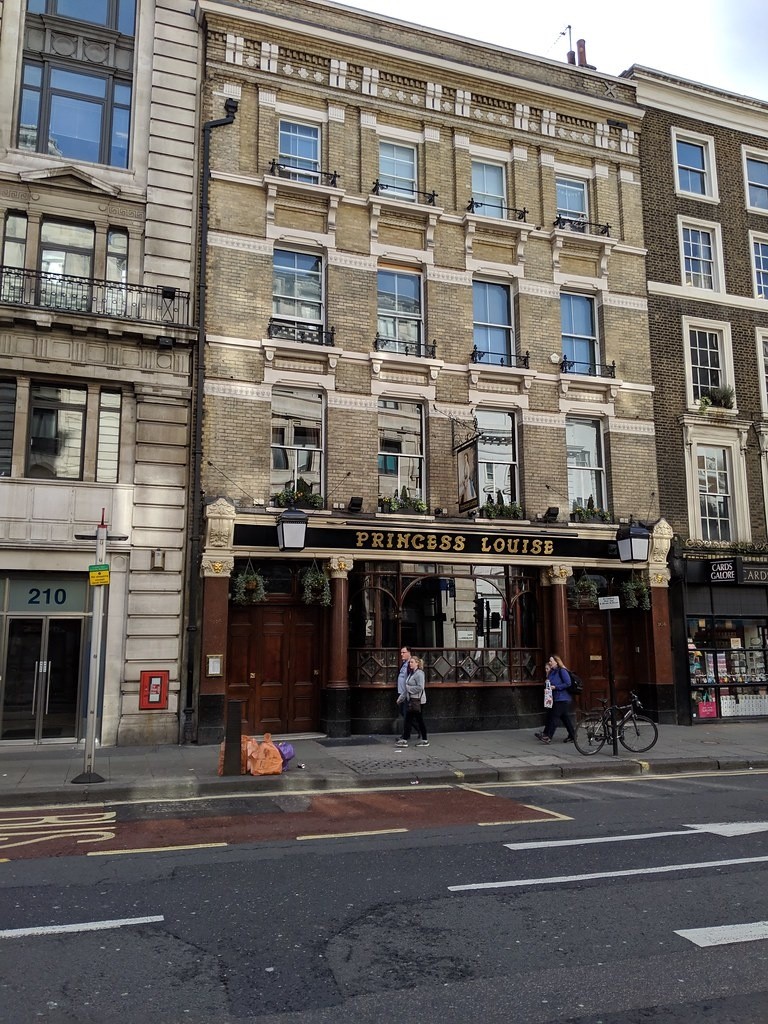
[396,645,421,741]
[394,656,430,747]
[462,453,477,501]
[538,655,578,744]
[534,662,552,739]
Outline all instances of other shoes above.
[395,736,405,741]
[563,738,574,743]
[417,732,421,739]
[535,732,551,744]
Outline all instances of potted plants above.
[620,575,652,613]
[569,578,598,608]
[231,566,270,605]
[292,566,333,608]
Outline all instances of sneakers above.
[415,740,430,746]
[395,740,409,747]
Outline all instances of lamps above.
[545,507,560,517]
[275,497,310,551]
[162,284,176,300]
[616,514,650,563]
[347,497,364,513]
[157,337,174,351]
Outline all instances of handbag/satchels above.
[408,698,421,713]
[544,682,553,708]
[698,692,716,718]
[558,668,584,696]
[218,733,295,775]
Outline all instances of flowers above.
[382,485,427,514]
[480,490,523,519]
[274,476,324,508]
[574,494,611,524]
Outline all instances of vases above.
[480,510,521,520]
[569,513,606,524]
[382,504,423,516]
[278,499,322,512]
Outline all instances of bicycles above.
[573,688,658,755]
